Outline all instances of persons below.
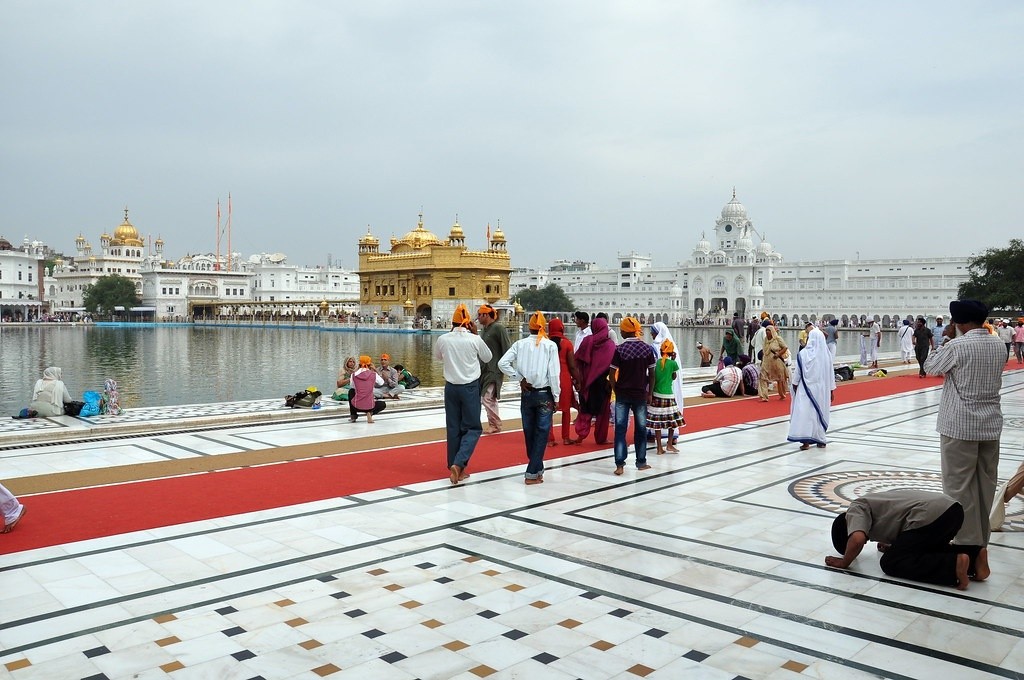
[99,379,121,415]
[348,356,387,423]
[29,366,73,417]
[373,354,405,399]
[393,365,413,389]
[477,305,511,433]
[548,317,582,446]
[645,322,684,445]
[923,300,1009,547]
[1004,463,1024,503]
[572,319,614,444]
[825,490,991,590]
[787,329,837,451]
[0,484,27,533]
[574,310,592,355]
[332,355,359,400]
[1,309,447,329]
[608,317,657,474]
[435,306,493,484]
[680,306,1024,402]
[498,309,561,484]
[591,312,617,426]
[646,339,687,455]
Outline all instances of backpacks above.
[292,390,321,409]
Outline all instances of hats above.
[903,320,909,325]
[830,319,839,325]
[1003,319,1010,323]
[936,315,943,320]
[949,300,988,325]
[918,318,926,324]
[995,319,1001,321]
[832,512,848,555]
[865,316,874,323]
[989,319,993,324]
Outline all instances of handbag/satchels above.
[79,391,102,416]
